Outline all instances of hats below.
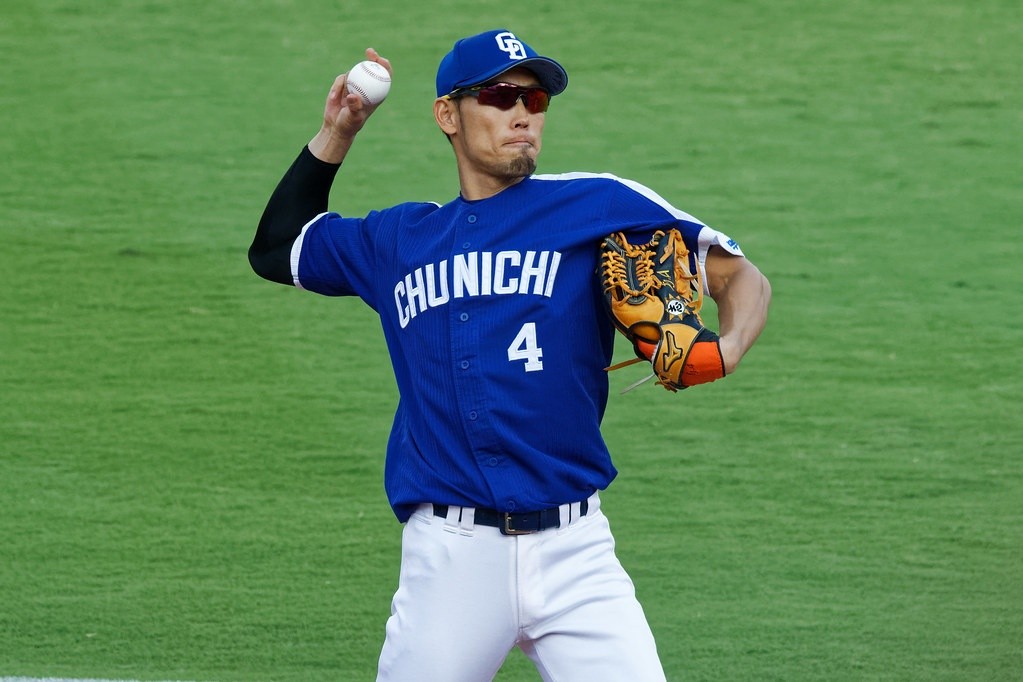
[435,28,568,97]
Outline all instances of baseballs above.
[346,60,393,107]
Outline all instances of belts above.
[434,499,588,534]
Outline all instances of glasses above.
[436,81,551,114]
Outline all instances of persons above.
[246,27,773,682]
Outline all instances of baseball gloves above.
[598,227,706,393]
